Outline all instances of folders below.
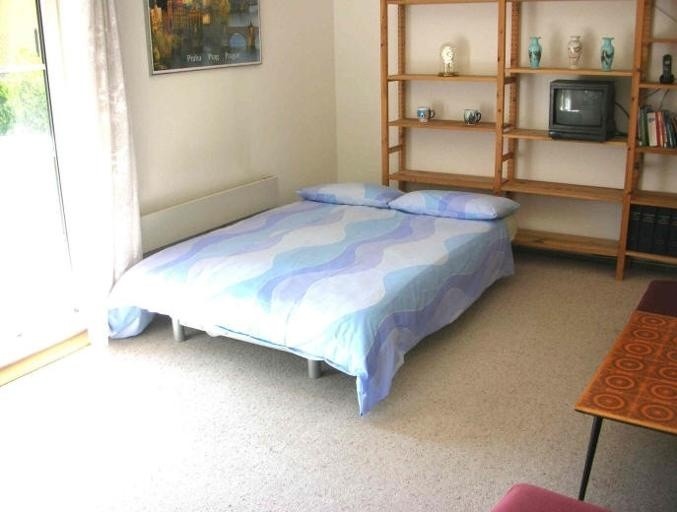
[627,204,677,257]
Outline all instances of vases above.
[566,36,582,70]
[598,36,615,71]
[525,35,544,69]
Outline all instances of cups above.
[417,107,435,123]
[463,109,482,125]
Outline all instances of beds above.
[141,180,518,378]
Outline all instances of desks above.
[573,278,676,501]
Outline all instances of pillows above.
[296,180,520,222]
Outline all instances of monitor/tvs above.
[549,80,615,141]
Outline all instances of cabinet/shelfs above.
[378,1,676,282]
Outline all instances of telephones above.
[659,54,674,84]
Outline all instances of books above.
[638,105,677,149]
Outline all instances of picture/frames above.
[144,0,262,76]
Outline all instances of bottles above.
[529,35,615,71]
